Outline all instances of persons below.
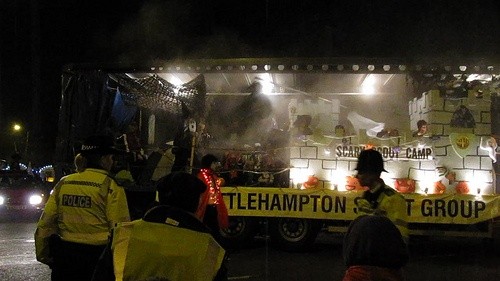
[170,104,195,172]
[356,149,409,252]
[102,172,228,281]
[413,120,440,141]
[35,132,131,281]
[338,214,404,281]
[196,155,229,235]
[74,153,88,173]
[434,163,470,195]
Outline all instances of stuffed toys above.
[304,158,333,194]
[390,174,417,192]
[346,170,365,191]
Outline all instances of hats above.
[354,150,389,176]
[155,172,207,212]
[81,128,125,155]
[200,154,221,168]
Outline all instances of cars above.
[0,168,46,213]
